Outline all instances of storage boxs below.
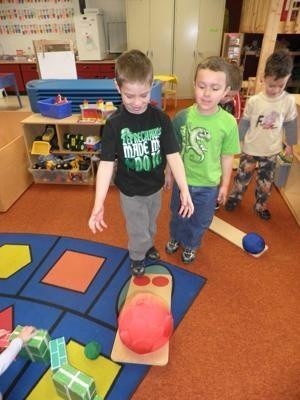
[28,159,92,186]
[1,110,36,212]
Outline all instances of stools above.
[153,75,178,112]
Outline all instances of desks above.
[0,72,23,108]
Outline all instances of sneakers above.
[146,246,160,261]
[165,238,180,255]
[130,258,146,276]
[225,197,240,212]
[181,248,197,264]
[253,204,272,219]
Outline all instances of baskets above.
[37,97,74,119]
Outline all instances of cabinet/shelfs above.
[0,56,26,96]
[20,55,39,86]
[20,113,116,186]
[238,0,300,88]
[76,62,117,79]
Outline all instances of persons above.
[224,50,298,221]
[162,56,242,263]
[88,49,195,278]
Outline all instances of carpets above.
[0,233,207,400]
